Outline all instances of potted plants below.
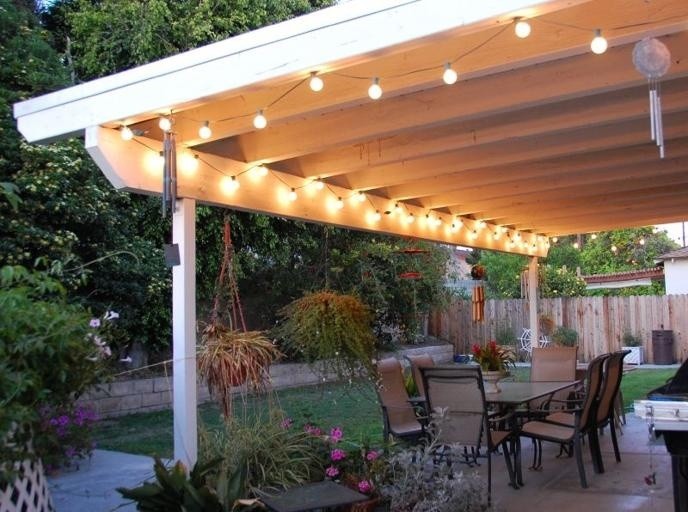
[195,215,284,431]
[619,331,645,365]
[273,293,378,404]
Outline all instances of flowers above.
[277,415,378,495]
[469,344,516,370]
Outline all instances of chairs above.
[517,349,631,490]
[529,347,579,380]
[418,364,514,509]
[376,355,435,465]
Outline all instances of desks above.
[409,381,581,456]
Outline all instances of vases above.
[481,370,512,395]
